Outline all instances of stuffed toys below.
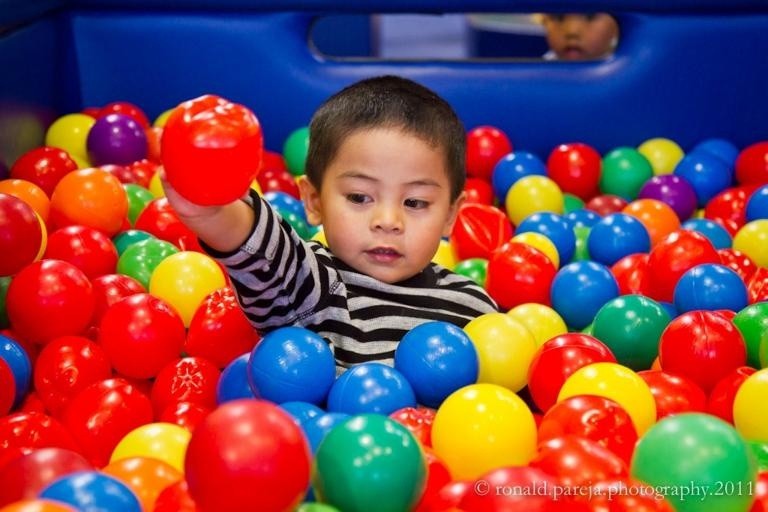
[0,94,768,511]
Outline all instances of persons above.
[0,94,768,511]
[159,76,502,380]
[532,15,616,61]
